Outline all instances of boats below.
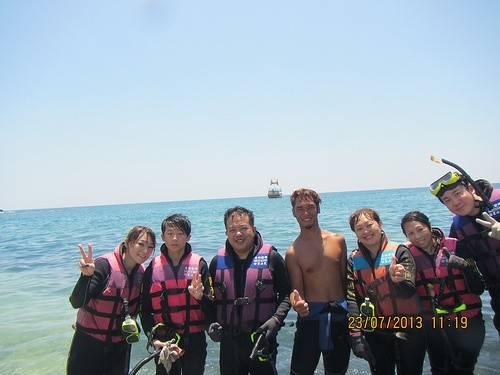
[267,179,282,198]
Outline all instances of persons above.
[202,205,293,375]
[66,225,157,375]
[140,213,214,375]
[344,209,423,375]
[401,211,487,375]
[286,189,351,375]
[429,155,500,338]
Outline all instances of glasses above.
[250,332,272,361]
[151,323,181,346]
[122,319,140,344]
[360,298,375,332]
[435,304,466,316]
[429,171,459,195]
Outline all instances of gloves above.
[208,322,223,342]
[475,212,500,240]
[442,247,467,270]
[351,336,376,367]
[257,316,281,343]
